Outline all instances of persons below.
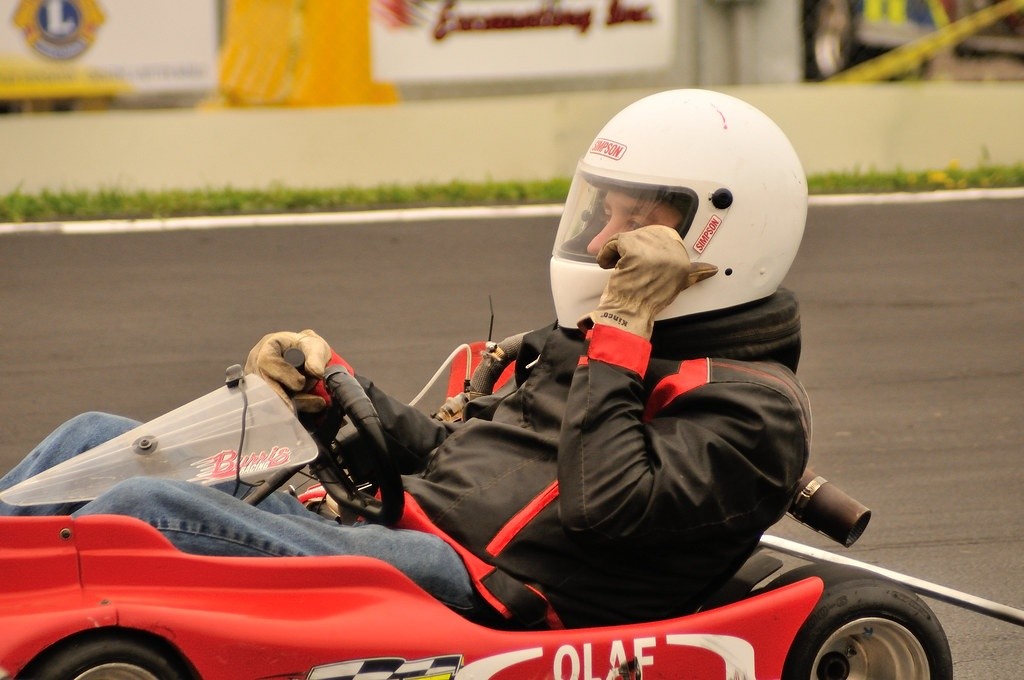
[0,88,812,632]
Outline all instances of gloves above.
[576,223,719,343]
[243,330,331,417]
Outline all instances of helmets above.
[549,87,808,329]
